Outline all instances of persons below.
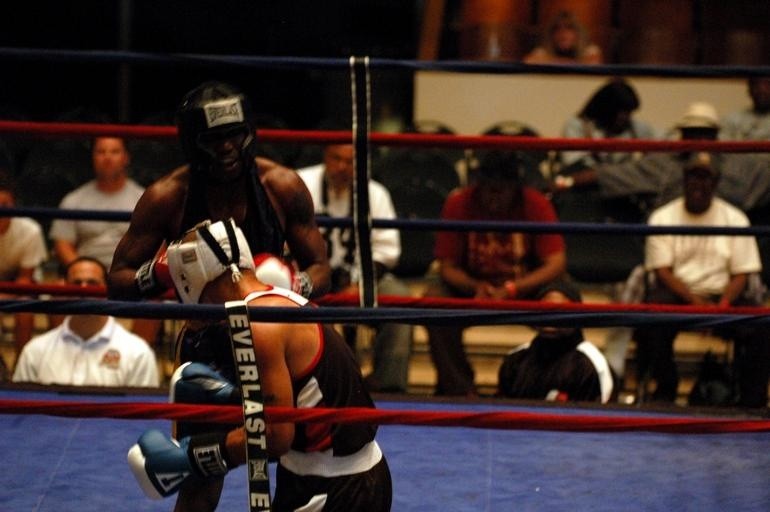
[295,141,415,393]
[12,257,161,390]
[109,78,334,511]
[631,152,769,407]
[0,180,51,374]
[50,136,146,276]
[419,149,566,394]
[127,214,393,512]
[555,65,770,220]
[496,279,619,406]
[525,9,602,67]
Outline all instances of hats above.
[673,101,721,129]
